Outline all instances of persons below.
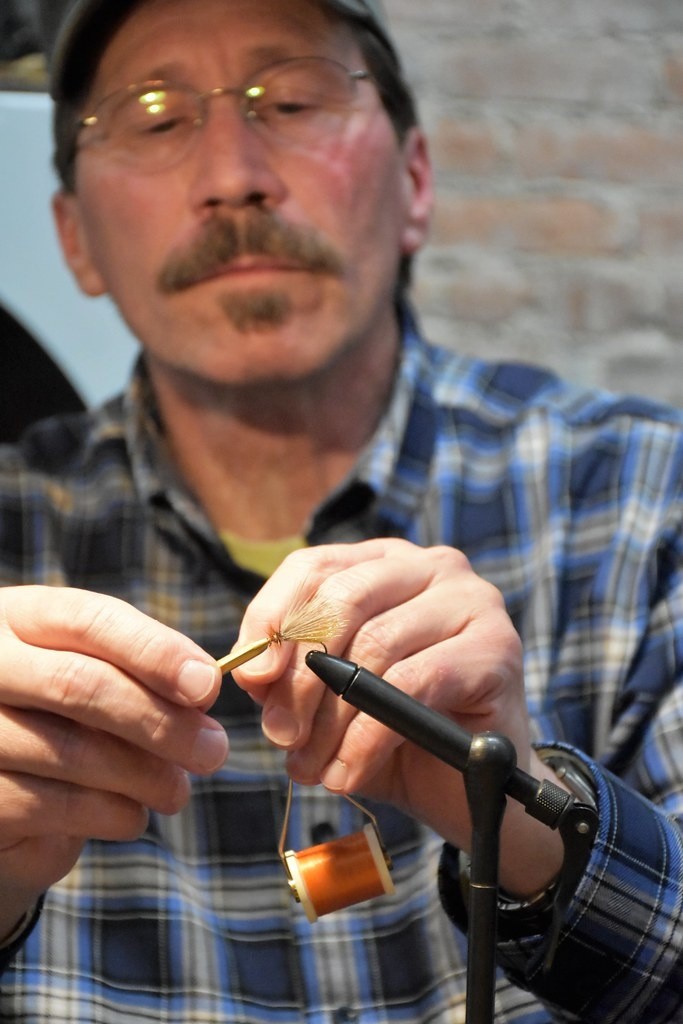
[0,1,683,1024]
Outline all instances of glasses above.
[79,56,373,176]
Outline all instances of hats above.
[45,0,403,104]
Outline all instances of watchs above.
[457,753,600,921]
